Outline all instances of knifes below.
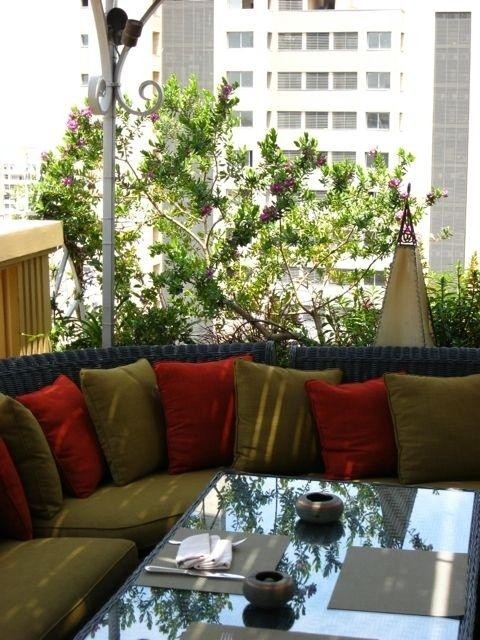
[144,566,246,580]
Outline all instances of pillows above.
[304,376,401,479]
[0,393,64,520]
[385,371,479,483]
[154,354,254,476]
[78,359,167,487]
[13,372,109,502]
[229,358,351,469]
[0,438,33,540]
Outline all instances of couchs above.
[0,340,276,640]
[290,347,480,488]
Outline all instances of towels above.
[176,533,232,570]
